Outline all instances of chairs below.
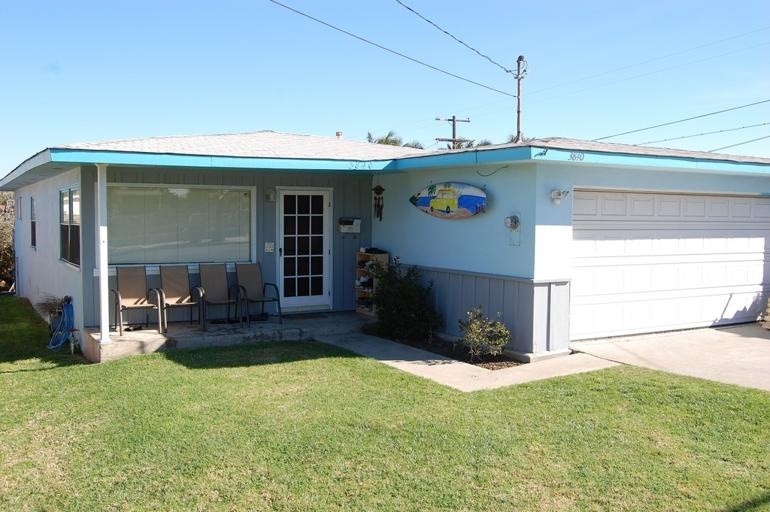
[155,265,205,333]
[234,262,282,327]
[110,265,163,336]
[198,263,243,331]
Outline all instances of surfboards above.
[409,182,486,219]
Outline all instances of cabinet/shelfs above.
[354,251,389,318]
